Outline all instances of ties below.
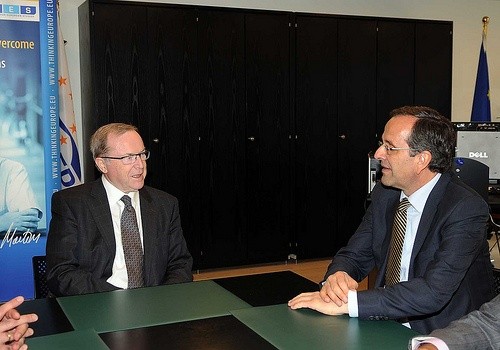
[120,195,145,288]
[385,197,410,286]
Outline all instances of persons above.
[407,289,500,350]
[46,122,193,297]
[286,105,496,336]
[0,156,43,235]
[0,296,38,350]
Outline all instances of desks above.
[485,177,500,236]
[1,268,428,349]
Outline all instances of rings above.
[7,332,11,342]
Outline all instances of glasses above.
[100,151,151,165]
[378,141,414,156]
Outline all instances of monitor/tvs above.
[457,131,500,183]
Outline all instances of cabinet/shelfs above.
[78,1,454,275]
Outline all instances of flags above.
[470,14,493,122]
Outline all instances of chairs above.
[486,267,500,301]
[451,156,489,207]
[32,255,49,300]
